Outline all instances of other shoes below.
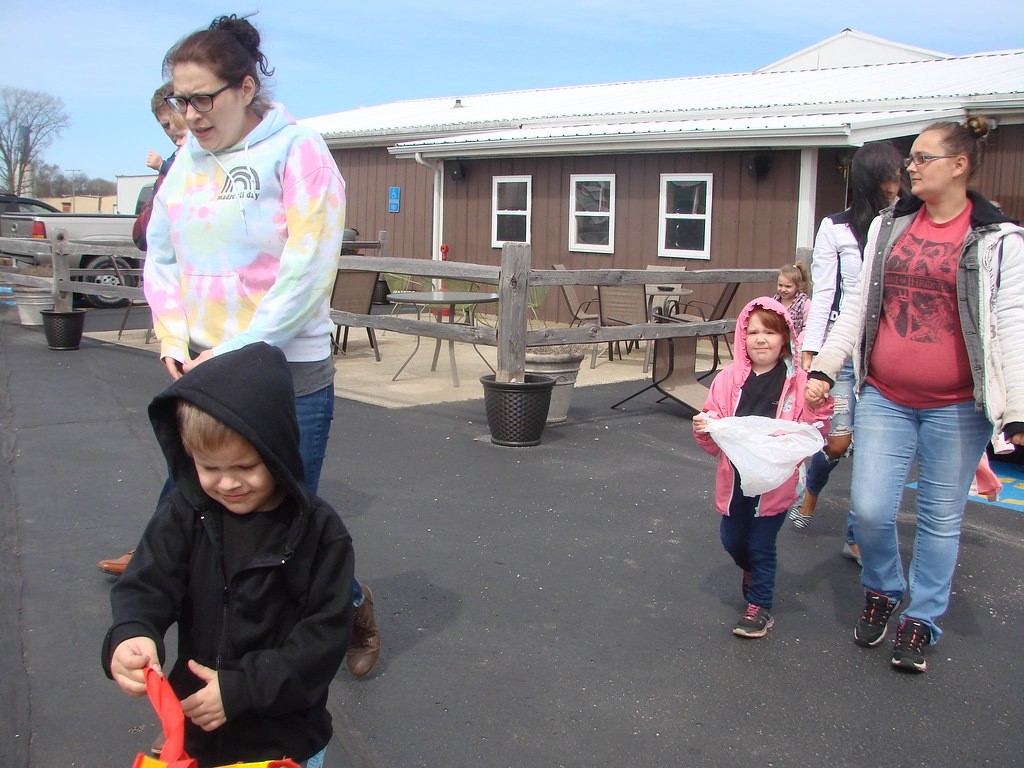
[842,543,867,566]
[788,503,814,530]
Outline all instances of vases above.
[39,309,88,350]
[480,374,556,446]
[524,347,586,421]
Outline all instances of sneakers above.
[741,570,753,602]
[853,592,901,645]
[733,604,774,638]
[891,615,929,672]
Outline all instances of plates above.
[657,286,675,291]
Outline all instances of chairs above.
[107,254,153,344]
[330,264,741,415]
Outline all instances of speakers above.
[449,164,465,180]
[747,163,763,177]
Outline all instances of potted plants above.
[12,264,55,326]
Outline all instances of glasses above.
[903,153,958,167]
[163,75,245,113]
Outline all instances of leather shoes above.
[96,547,137,574]
[344,584,381,676]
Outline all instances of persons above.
[804,119,1024,669]
[774,261,811,344]
[99,81,188,574]
[143,17,380,677]
[102,343,355,768]
[692,297,835,638]
[789,143,903,566]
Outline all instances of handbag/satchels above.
[695,409,825,496]
[132,669,302,768]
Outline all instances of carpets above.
[905,461,1024,512]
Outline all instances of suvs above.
[0,193,62,214]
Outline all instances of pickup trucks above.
[0,182,156,309]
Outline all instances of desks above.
[646,285,694,308]
[387,290,499,387]
